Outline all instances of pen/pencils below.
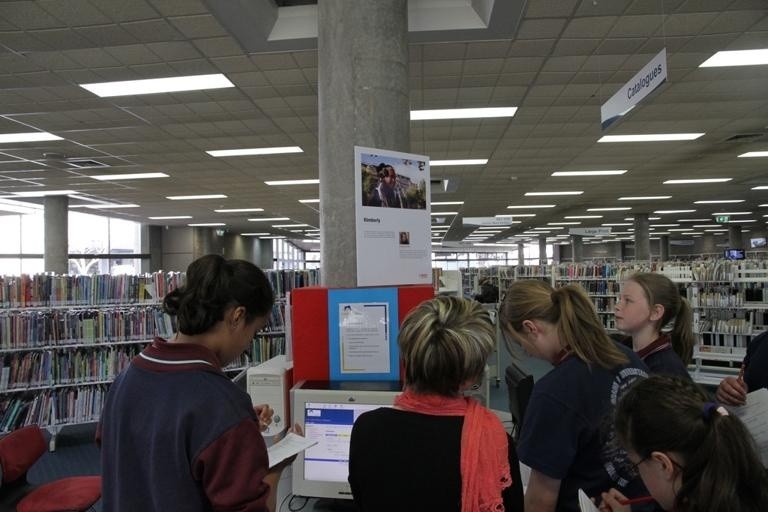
[599,496,654,510]
[737,364,746,383]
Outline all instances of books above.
[718,386,767,471]
[262,425,320,470]
[577,486,602,512]
[1,271,187,433]
[221,268,321,371]
[455,255,768,357]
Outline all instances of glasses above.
[616,448,686,482]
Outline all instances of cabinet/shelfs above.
[0,256,767,451]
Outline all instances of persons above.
[349,294,526,510]
[577,372,768,511]
[365,162,406,208]
[400,232,408,245]
[95,252,305,511]
[613,270,697,386]
[496,278,665,512]
[714,329,768,409]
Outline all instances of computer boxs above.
[246,354,295,446]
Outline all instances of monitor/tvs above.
[290,388,403,500]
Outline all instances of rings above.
[719,379,727,387]
